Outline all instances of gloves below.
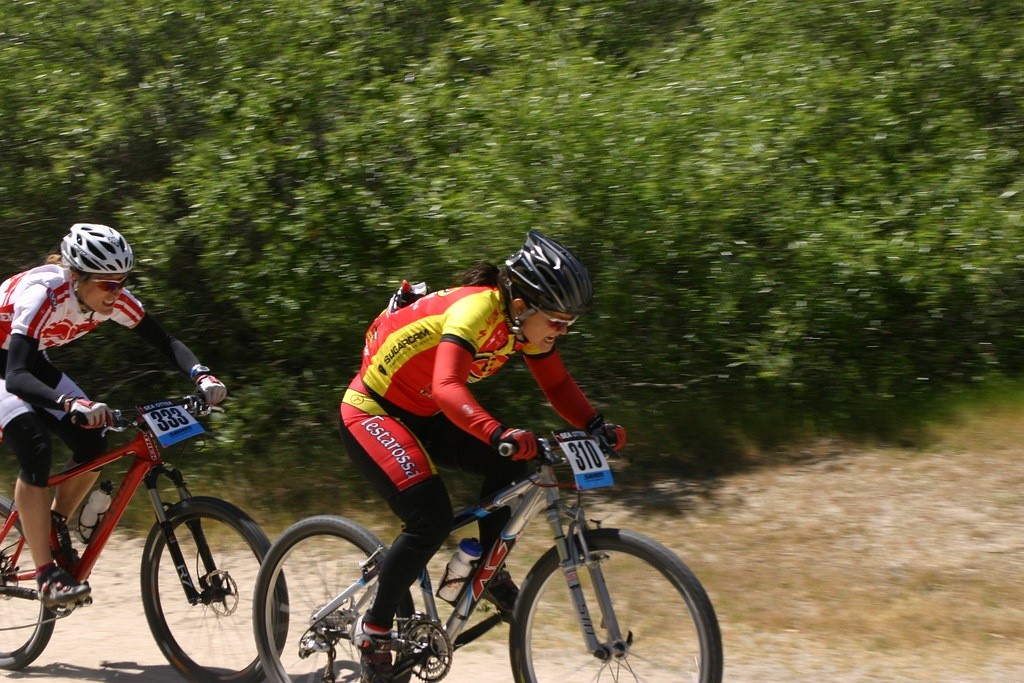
[585,412,626,453]
[488,424,537,461]
[196,374,226,407]
[69,396,113,428]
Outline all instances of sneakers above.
[35,566,91,608]
[49,510,80,569]
[349,613,393,683]
[481,563,519,612]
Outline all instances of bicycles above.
[252,428,724,683]
[0,381,292,683]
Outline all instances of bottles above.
[74,479,114,544]
[439,537,483,602]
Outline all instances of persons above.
[0,223,226,607]
[340,229,626,683]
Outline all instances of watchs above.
[193,366,210,378]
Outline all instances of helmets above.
[61,223,134,273]
[506,229,592,315]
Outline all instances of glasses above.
[526,301,579,329]
[92,274,128,291]
